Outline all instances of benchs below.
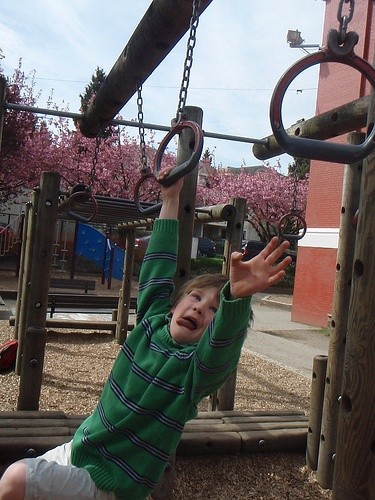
[48,297,140,317]
[48,279,99,295]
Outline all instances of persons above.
[0,164,292,500]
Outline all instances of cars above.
[198,233,261,263]
